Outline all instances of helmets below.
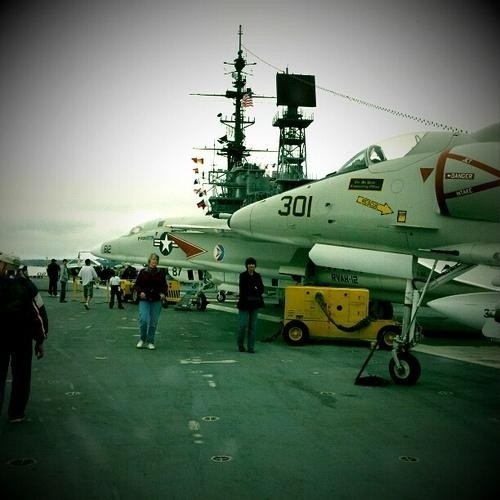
[0,248,20,266]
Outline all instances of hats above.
[51,259,57,262]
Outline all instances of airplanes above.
[0,249,24,268]
[66,122,500,385]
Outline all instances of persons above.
[0,253,138,423]
[237,257,264,353]
[134,254,168,350]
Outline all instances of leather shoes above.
[239,346,255,353]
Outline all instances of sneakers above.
[136,340,155,349]
[84,302,90,308]
[10,417,26,422]
